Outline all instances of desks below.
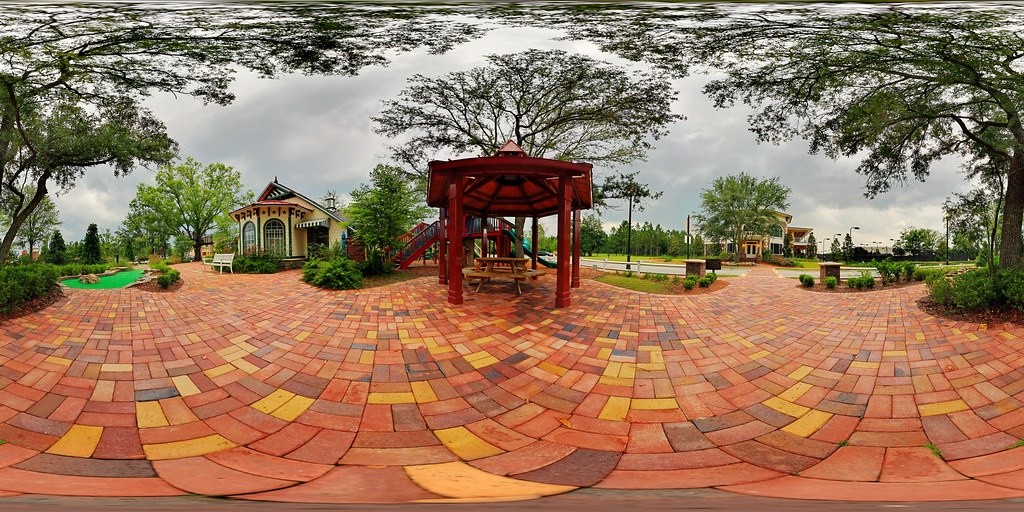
[464,258,537,294]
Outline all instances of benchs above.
[201,252,235,275]
[462,267,548,280]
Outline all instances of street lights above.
[850,226,859,242]
[823,238,830,262]
[833,234,841,239]
[873,242,882,249]
[817,241,822,244]
[890,239,900,244]
[860,244,868,249]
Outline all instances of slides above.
[504,229,557,268]
